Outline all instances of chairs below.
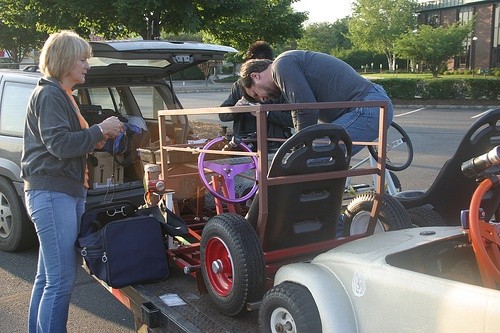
[394,108,500,226]
[245,124,353,263]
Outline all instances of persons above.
[219,41,294,147]
[19,30,125,333]
[240,50,393,165]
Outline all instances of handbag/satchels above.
[75,200,188,289]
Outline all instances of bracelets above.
[98,124,103,132]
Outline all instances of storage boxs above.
[88,151,124,188]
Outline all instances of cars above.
[138,100,387,316]
[394,106,500,229]
[257,145,500,333]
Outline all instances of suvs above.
[0,40,240,253]
